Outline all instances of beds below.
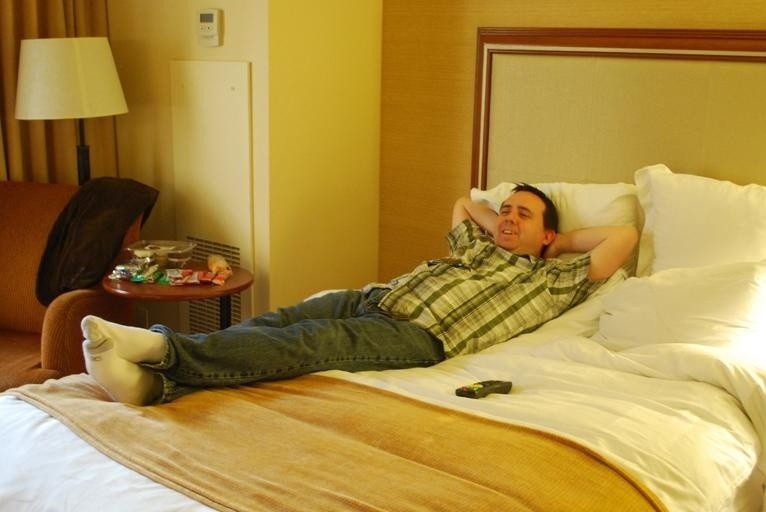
[0,23,764,511]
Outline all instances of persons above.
[79,182,639,408]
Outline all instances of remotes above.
[456,381,512,399]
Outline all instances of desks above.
[101,262,254,334]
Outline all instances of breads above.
[206,254,232,273]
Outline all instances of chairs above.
[0,179,147,391]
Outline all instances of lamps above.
[11,34,131,184]
